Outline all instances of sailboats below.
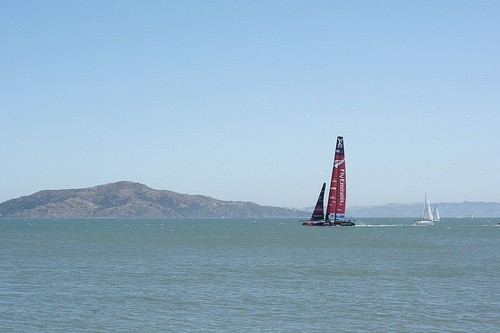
[302,136,356,226]
[415,195,441,225]
